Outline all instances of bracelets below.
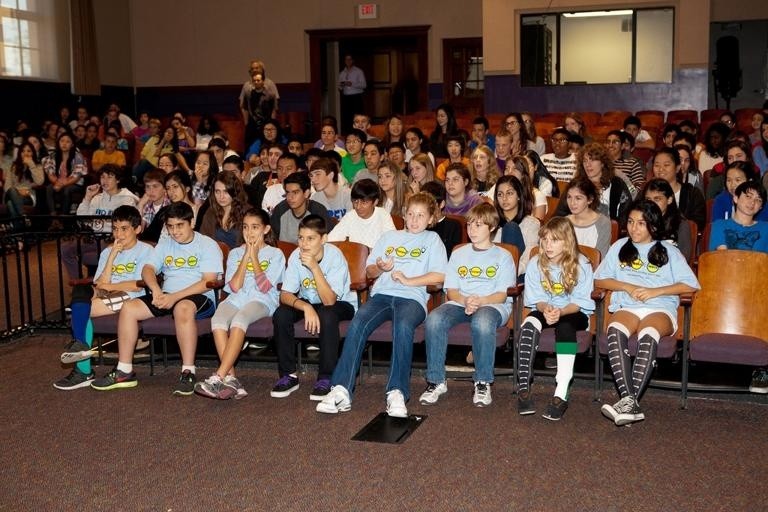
[291,298,299,307]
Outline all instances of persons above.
[708,183,767,395]
[316,193,449,419]
[195,209,285,403]
[466,176,540,363]
[592,201,701,429]
[545,177,612,369]
[0,104,768,268]
[514,218,594,421]
[270,214,360,400]
[200,173,251,351]
[239,61,280,151]
[50,206,155,392]
[337,55,366,135]
[419,201,516,409]
[90,202,223,394]
[249,175,330,352]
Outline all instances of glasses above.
[354,120,367,125]
[497,191,515,198]
[606,140,621,145]
[551,137,568,143]
[444,177,464,183]
[264,128,277,133]
[321,131,334,136]
[505,121,517,127]
[288,146,302,151]
[725,120,732,125]
[345,138,362,145]
[470,154,487,162]
[524,119,532,124]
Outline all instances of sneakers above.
[306,344,320,350]
[61,338,92,364]
[91,367,138,391]
[270,375,300,398]
[386,389,408,418]
[541,396,568,421]
[472,381,492,408]
[249,342,266,350]
[749,368,768,393]
[601,396,624,421]
[518,392,536,415]
[194,375,224,399]
[241,341,249,350]
[52,366,96,390]
[218,375,248,400]
[310,379,331,401]
[173,369,196,396]
[615,396,645,425]
[419,381,448,405]
[316,384,352,414]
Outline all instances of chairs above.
[687,220,698,268]
[546,196,560,221]
[236,242,297,339]
[429,243,519,395]
[140,241,230,377]
[689,249,768,394]
[431,215,467,253]
[631,147,656,166]
[702,223,710,253]
[704,197,713,223]
[132,112,308,154]
[702,169,714,197]
[356,272,428,386]
[390,215,404,231]
[67,242,157,376]
[519,245,606,401]
[557,180,568,197]
[294,242,368,387]
[592,266,694,410]
